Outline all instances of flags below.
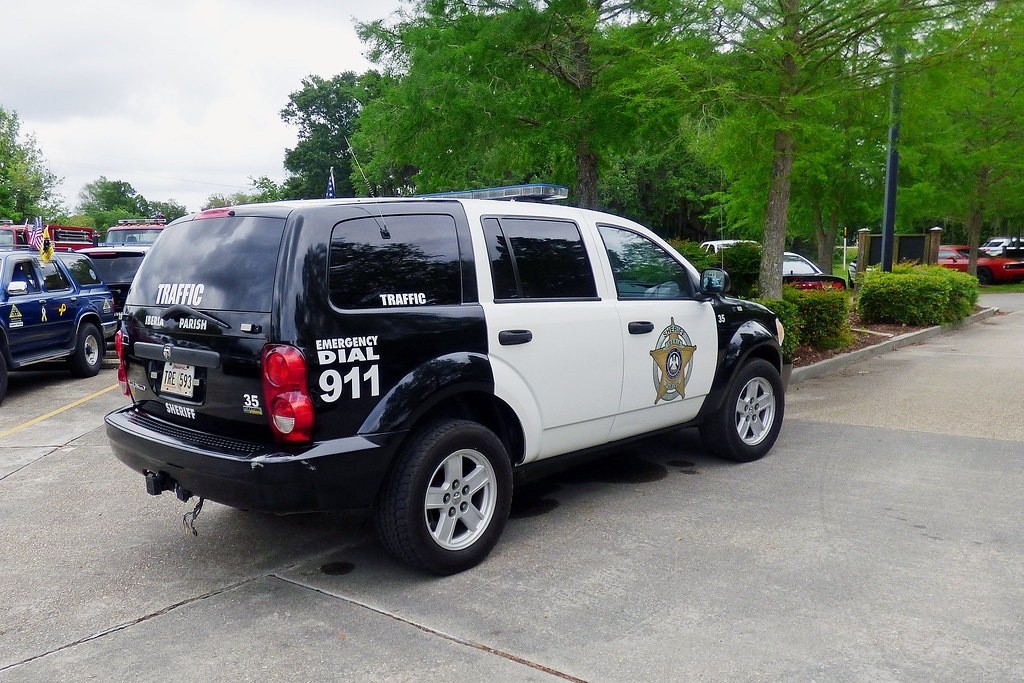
[326,166,335,199]
[23,216,53,263]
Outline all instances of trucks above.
[0,214,168,253]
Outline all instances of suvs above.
[977,236,1024,256]
[72,246,151,343]
[103,184,786,579]
[0,251,120,404]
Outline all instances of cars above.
[699,239,846,291]
[938,245,1024,284]
